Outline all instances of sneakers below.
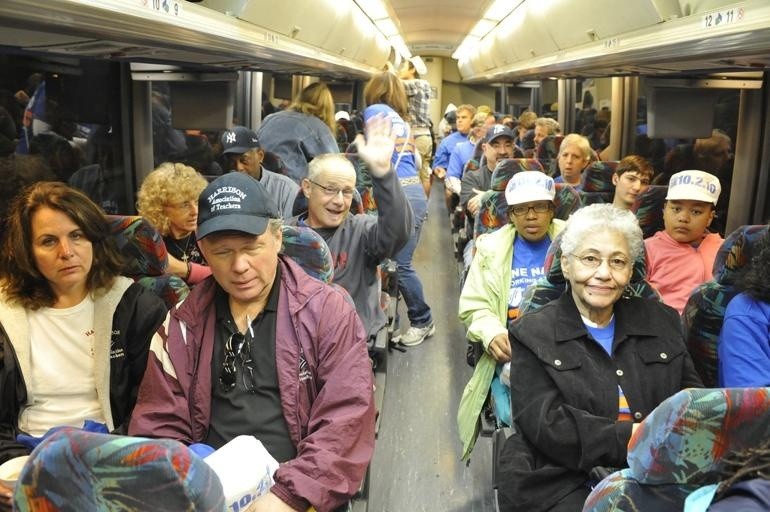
[397,320,436,347]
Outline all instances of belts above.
[399,175,421,187]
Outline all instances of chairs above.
[1,99,770,511]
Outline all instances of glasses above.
[569,253,633,270]
[479,111,494,128]
[309,179,353,194]
[219,332,256,395]
[509,204,549,216]
[162,200,198,209]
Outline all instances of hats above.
[334,111,350,122]
[195,168,280,242]
[505,170,557,205]
[485,123,516,143]
[221,125,260,156]
[665,169,722,207]
[408,55,426,75]
